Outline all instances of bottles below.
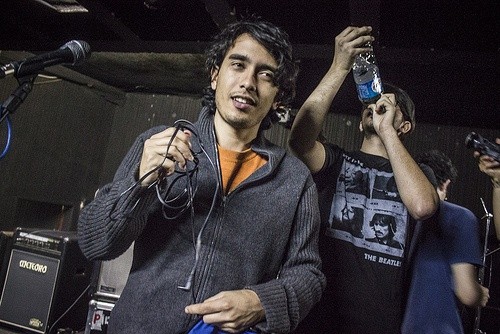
[353,40,386,116]
[462,132,500,166]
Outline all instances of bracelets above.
[491,178,500,189]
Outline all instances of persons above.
[77,16,325,334]
[401,147,491,334]
[473,138,500,241]
[289,25,440,334]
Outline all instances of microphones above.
[0,39,92,79]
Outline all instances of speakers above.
[0,226,94,334]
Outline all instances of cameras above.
[464,131,500,163]
